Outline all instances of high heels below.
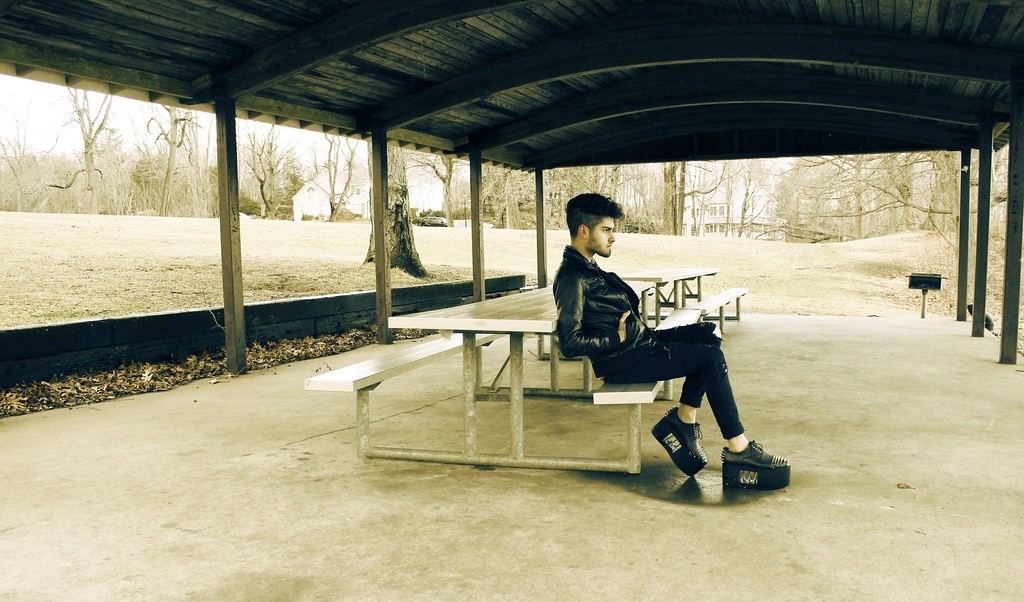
[721,440,790,490]
[652,407,708,477]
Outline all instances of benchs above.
[309,333,508,453]
[684,287,749,333]
[592,309,703,472]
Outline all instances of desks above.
[618,268,718,322]
[388,281,658,468]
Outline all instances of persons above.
[552,193,791,489]
